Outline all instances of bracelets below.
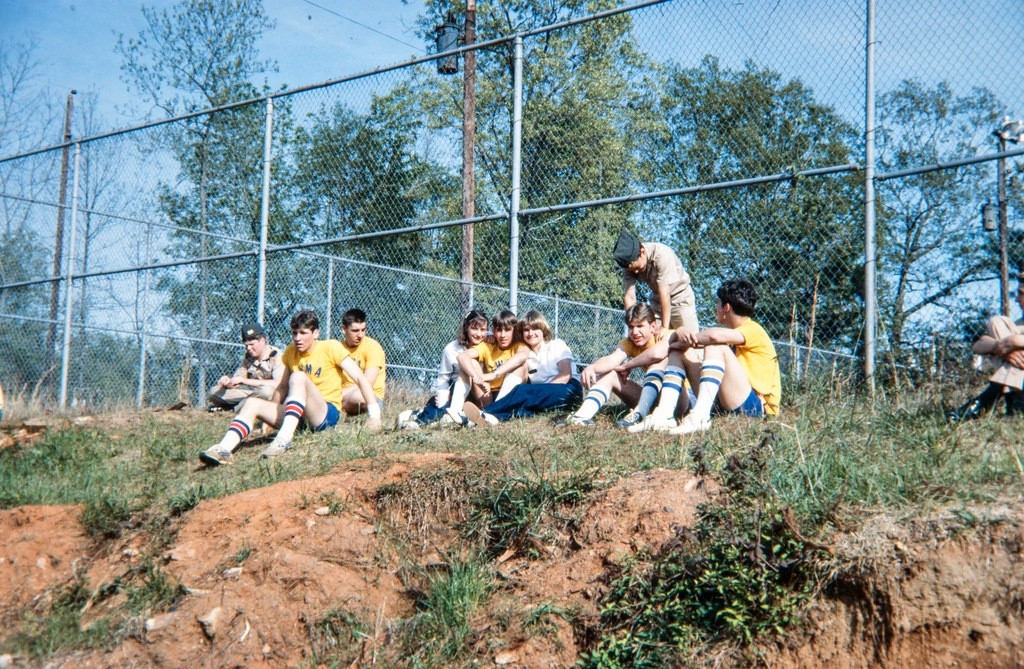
[494,371,500,378]
[367,403,381,418]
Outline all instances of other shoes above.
[555,412,595,426]
[615,408,643,427]
[627,406,678,432]
[946,397,984,427]
[446,407,476,428]
[398,409,421,432]
[464,401,494,428]
[669,411,712,436]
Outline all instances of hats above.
[613,225,640,268]
[241,322,265,340]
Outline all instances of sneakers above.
[259,440,292,459]
[199,444,235,465]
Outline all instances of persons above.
[947,271,1024,424]
[394,309,583,432]
[553,232,782,435]
[198,309,386,466]
